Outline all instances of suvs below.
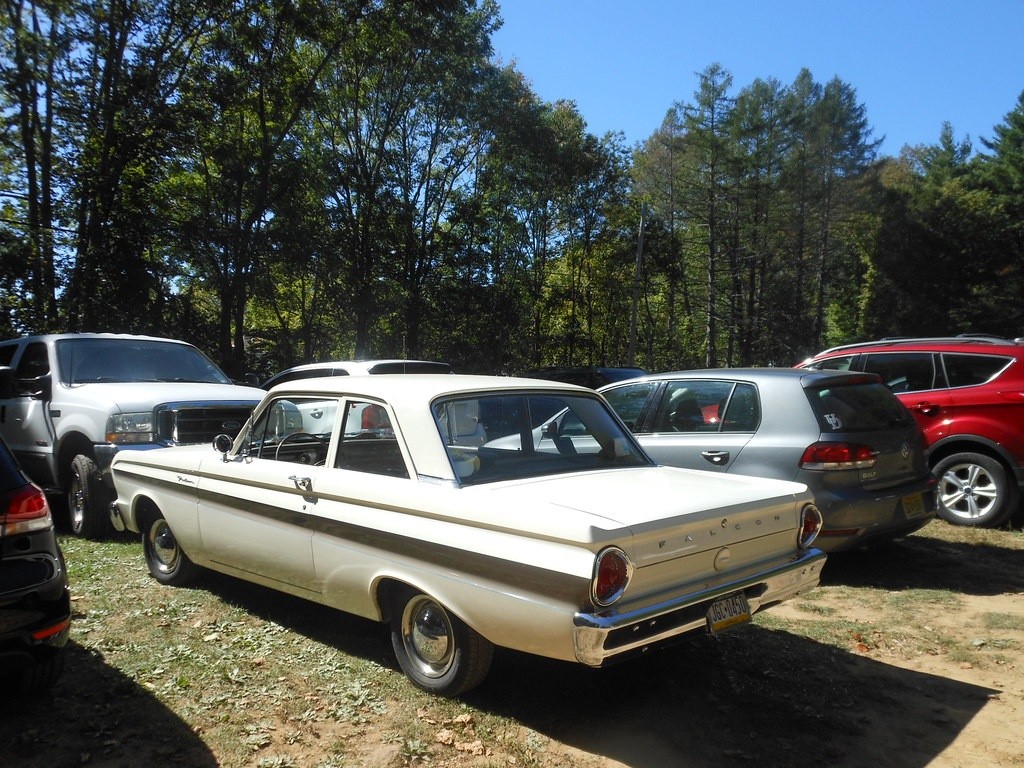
[0,332,304,540]
[700,337,1024,527]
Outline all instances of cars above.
[483,367,939,554]
[0,436,73,672]
[478,366,648,426]
[111,374,828,694]
[256,358,488,448]
